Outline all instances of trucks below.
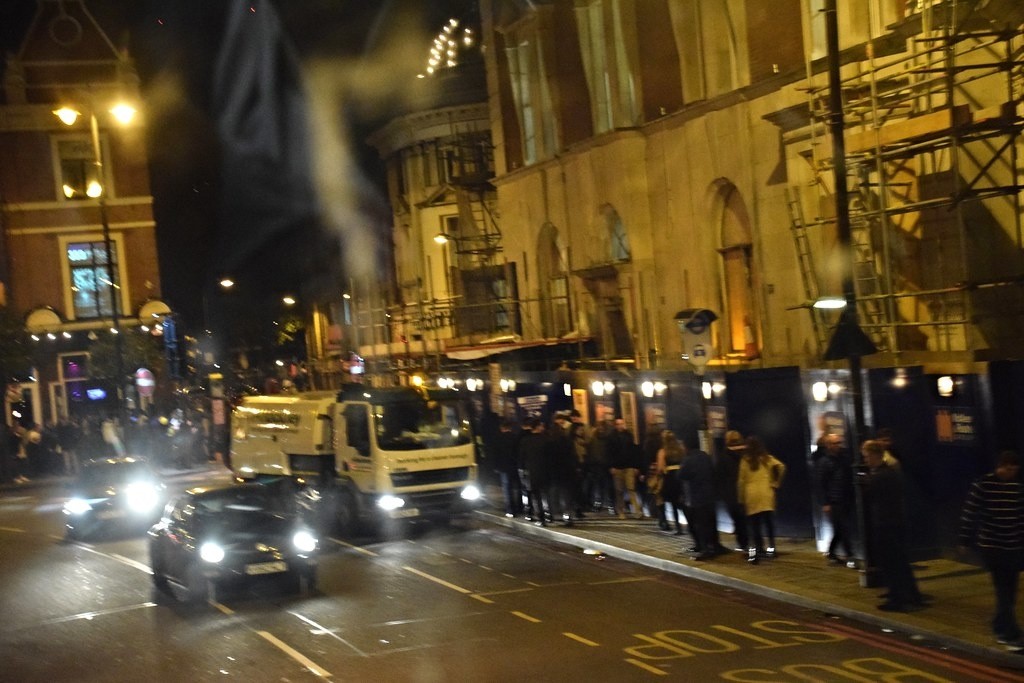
[230,389,477,539]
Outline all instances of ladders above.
[782,184,833,362]
[844,167,891,353]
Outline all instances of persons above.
[857,425,921,613]
[261,363,280,394]
[0,405,226,484]
[813,436,854,569]
[958,450,1024,653]
[465,411,787,567]
[817,411,850,445]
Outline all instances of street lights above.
[53,97,136,419]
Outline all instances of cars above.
[63,456,163,537]
[148,482,320,604]
